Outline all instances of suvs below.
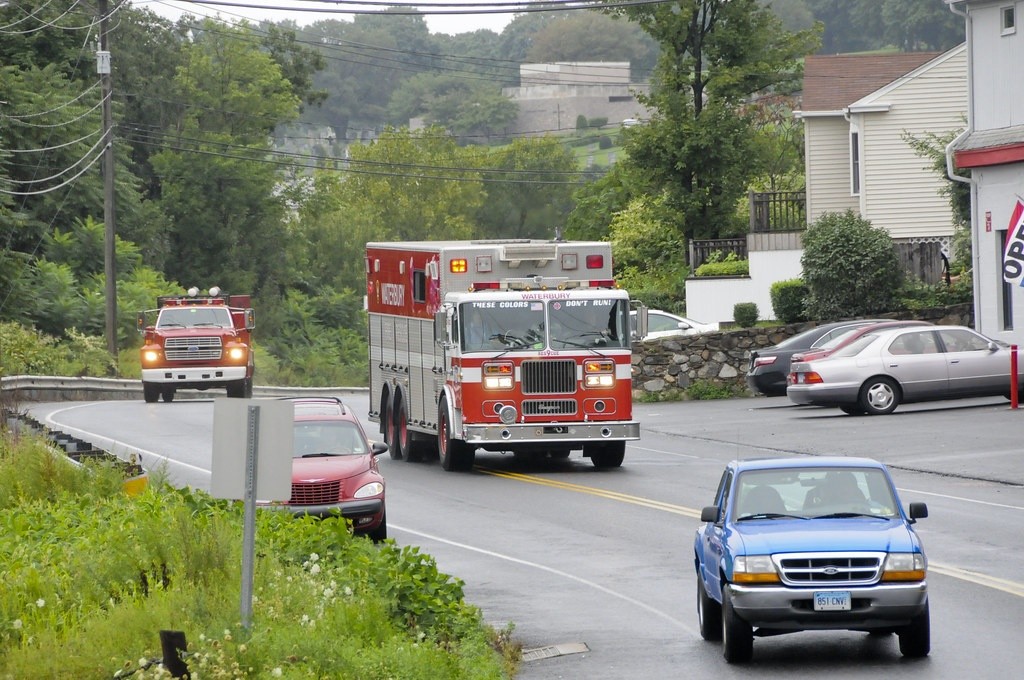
[692,455,931,664]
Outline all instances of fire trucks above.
[363,225,650,472]
[136,286,257,403]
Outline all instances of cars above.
[744,318,897,399]
[785,325,1024,416]
[790,321,961,375]
[619,310,721,342]
[252,395,389,546]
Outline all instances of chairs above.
[802,485,865,511]
[816,472,859,503]
[904,339,925,354]
[747,486,787,512]
